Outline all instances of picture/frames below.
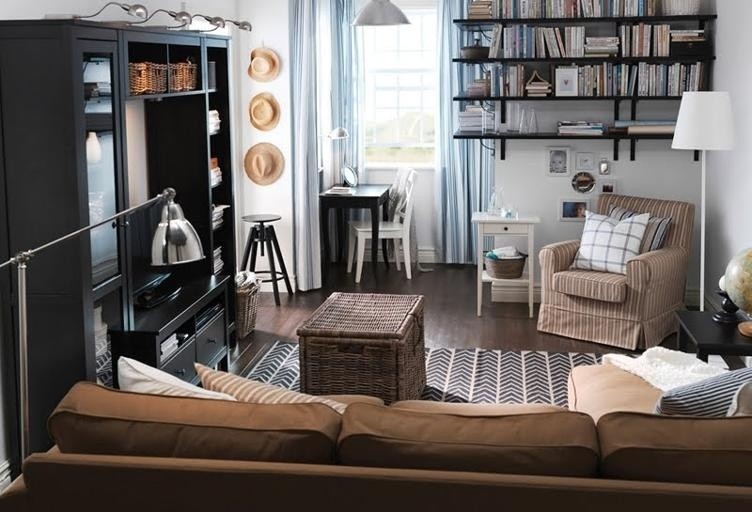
[598,159,612,176]
[559,198,590,222]
[572,173,596,194]
[599,179,616,194]
[574,151,596,172]
[546,146,571,175]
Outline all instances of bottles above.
[489,184,505,208]
[528,108,539,134]
[519,108,529,136]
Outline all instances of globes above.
[723,247,752,339]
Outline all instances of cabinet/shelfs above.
[109,274,232,389]
[0,19,130,480]
[122,30,207,102]
[205,27,237,325]
[453,12,717,162]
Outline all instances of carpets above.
[240,340,644,412]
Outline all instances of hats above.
[248,93,282,132]
[244,143,285,186]
[248,47,281,82]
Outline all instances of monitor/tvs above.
[129,206,191,311]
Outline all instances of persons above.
[550,150,567,173]
[573,203,586,217]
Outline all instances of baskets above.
[237,278,262,338]
[661,0,700,15]
[298,292,427,407]
[483,251,528,279]
[128,62,147,96]
[145,63,166,95]
[170,64,197,91]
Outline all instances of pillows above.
[607,203,672,255]
[118,356,234,402]
[570,210,650,276]
[655,368,752,419]
[194,362,347,415]
[725,381,752,422]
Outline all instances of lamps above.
[202,19,251,33]
[350,0,412,26]
[130,8,193,26]
[168,14,224,29]
[71,2,148,23]
[672,92,733,313]
[0,187,206,470]
[320,128,349,169]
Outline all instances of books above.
[236,270,257,289]
[489,21,705,59]
[457,106,494,132]
[210,157,224,188]
[454,1,705,18]
[209,108,222,136]
[214,244,225,273]
[608,117,676,139]
[160,328,189,363]
[556,120,605,136]
[467,62,705,97]
[212,202,230,231]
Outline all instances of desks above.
[471,212,540,319]
[672,308,751,364]
[320,183,394,284]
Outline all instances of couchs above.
[536,194,695,349]
[0,347,752,511]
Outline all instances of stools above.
[238,214,293,306]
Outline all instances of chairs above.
[347,169,421,282]
